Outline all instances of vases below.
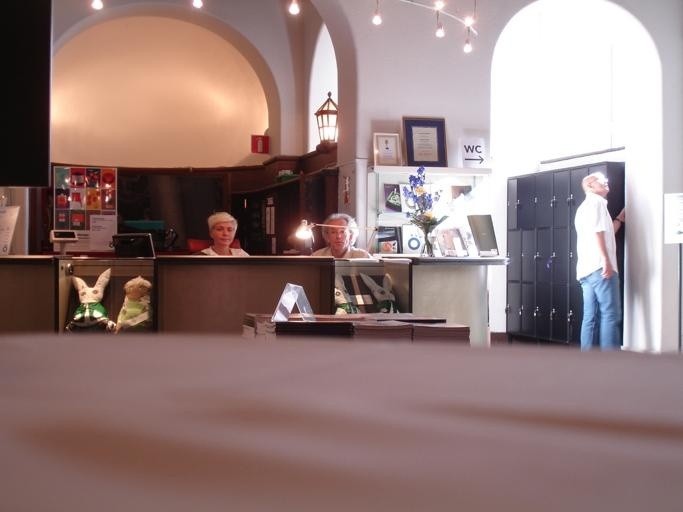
[421,225,435,257]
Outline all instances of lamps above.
[292,218,378,257]
[314,91,338,143]
[368,2,483,55]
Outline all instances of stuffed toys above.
[332,272,361,315]
[114,275,157,332]
[358,270,401,314]
[68,267,115,333]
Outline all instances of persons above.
[572,171,624,351]
[188,211,252,257]
[309,213,372,258]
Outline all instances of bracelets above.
[614,217,624,225]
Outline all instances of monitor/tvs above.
[112,233,156,257]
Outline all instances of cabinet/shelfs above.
[232,169,337,255]
[507,162,624,349]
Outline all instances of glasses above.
[589,177,608,185]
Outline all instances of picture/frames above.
[401,117,447,166]
[373,133,401,164]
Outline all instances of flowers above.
[401,166,448,257]
